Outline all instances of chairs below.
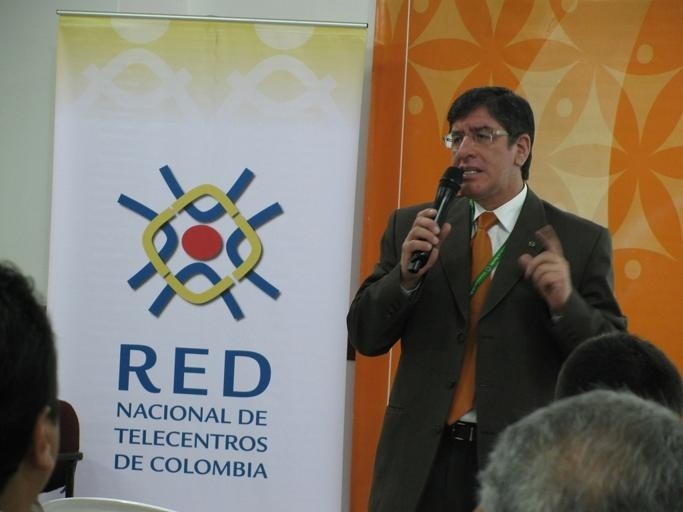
[40,399,83,498]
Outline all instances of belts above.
[444,419,478,443]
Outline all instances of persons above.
[471,390,681,512]
[345,86,627,512]
[552,330,683,417]
[0,262,60,511]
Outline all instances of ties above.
[446,210,500,426]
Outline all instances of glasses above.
[441,127,510,151]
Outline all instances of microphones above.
[407,166,465,275]
